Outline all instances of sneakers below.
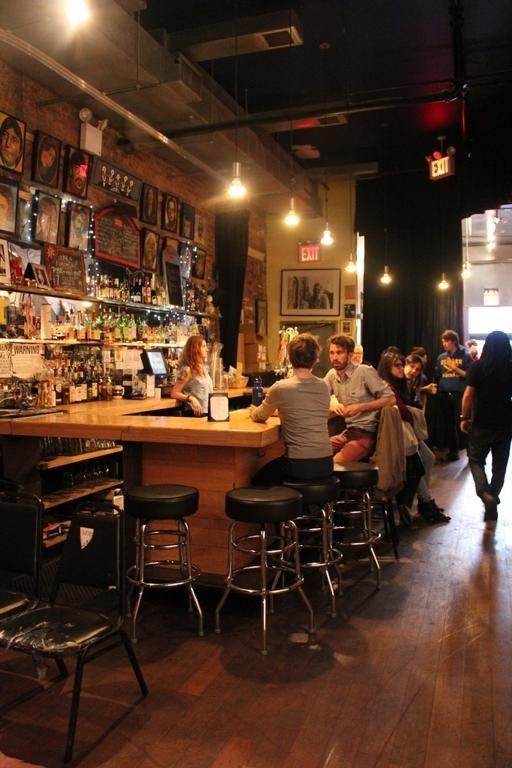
[483,508,499,522]
[478,488,501,505]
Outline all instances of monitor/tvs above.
[140,349,169,378]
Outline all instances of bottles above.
[251,374,265,410]
[180,240,199,278]
[432,366,440,389]
[21,301,213,348]
[0,350,114,410]
[186,276,209,314]
[88,259,168,309]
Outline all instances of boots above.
[398,505,420,531]
[416,495,451,524]
[439,446,459,461]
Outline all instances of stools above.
[123,482,207,644]
[215,483,315,659]
[327,460,383,594]
[268,477,348,620]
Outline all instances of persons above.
[170,334,214,416]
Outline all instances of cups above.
[112,384,125,400]
[38,435,117,496]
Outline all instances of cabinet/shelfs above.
[0,428,128,605]
[1,281,218,347]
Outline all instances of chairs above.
[2,497,151,766]
[1,488,69,680]
[332,403,406,562]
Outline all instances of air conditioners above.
[345,182,359,274]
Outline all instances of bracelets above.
[184,392,192,403]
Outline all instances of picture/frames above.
[140,182,195,240]
[0,177,20,237]
[142,231,208,280]
[2,111,26,175]
[63,146,91,199]
[280,268,342,316]
[2,239,88,294]
[30,130,62,188]
[91,152,139,206]
[31,190,62,246]
[65,201,94,254]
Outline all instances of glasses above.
[391,363,402,369]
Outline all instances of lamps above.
[320,166,336,248]
[378,219,394,286]
[436,220,452,293]
[229,14,248,204]
[283,92,300,228]
